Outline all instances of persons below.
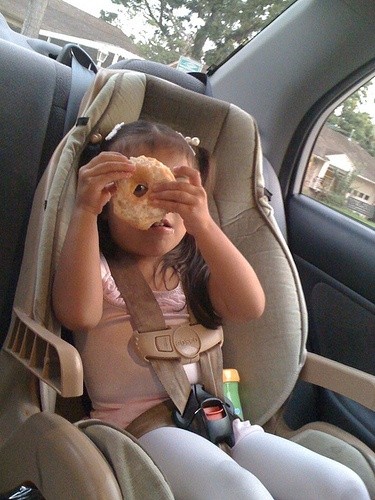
[50,118,372,500]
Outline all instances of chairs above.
[1,67,375,498]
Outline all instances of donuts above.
[111,155,176,230]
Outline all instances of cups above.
[217,367,246,421]
